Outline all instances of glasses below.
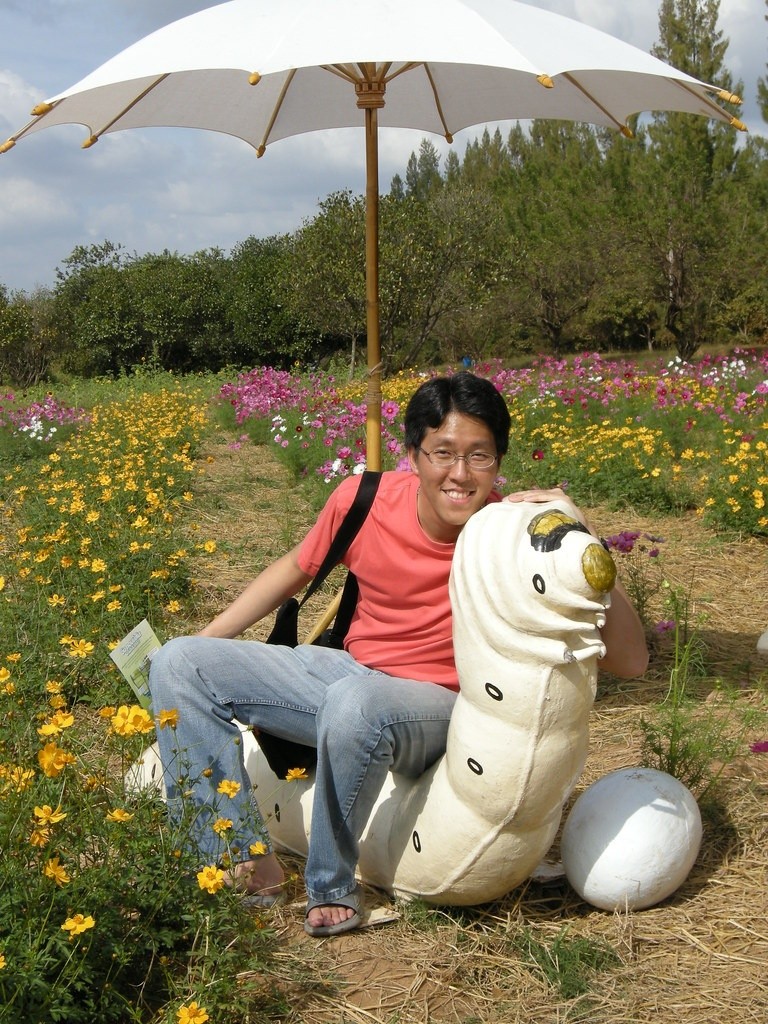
[415,445,500,469]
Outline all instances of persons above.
[149,371,650,938]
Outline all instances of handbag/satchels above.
[249,595,337,778]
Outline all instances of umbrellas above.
[0,0,749,473]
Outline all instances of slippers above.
[303,884,367,935]
[216,877,289,907]
